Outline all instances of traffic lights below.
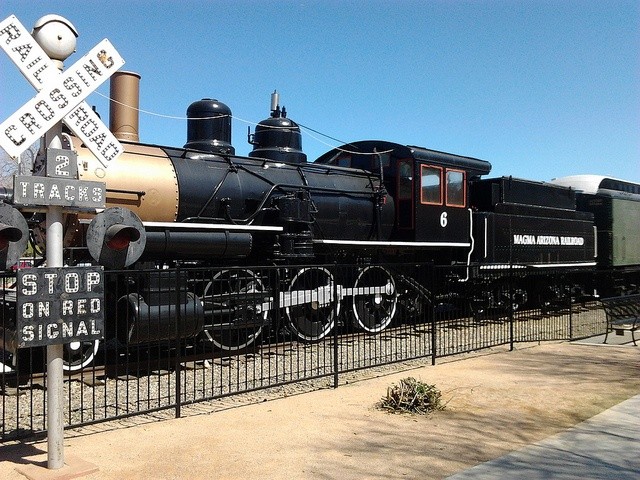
[0,203,29,270]
[85,207,147,269]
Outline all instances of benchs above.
[599,294,640,346]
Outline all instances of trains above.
[0,71,639,376]
